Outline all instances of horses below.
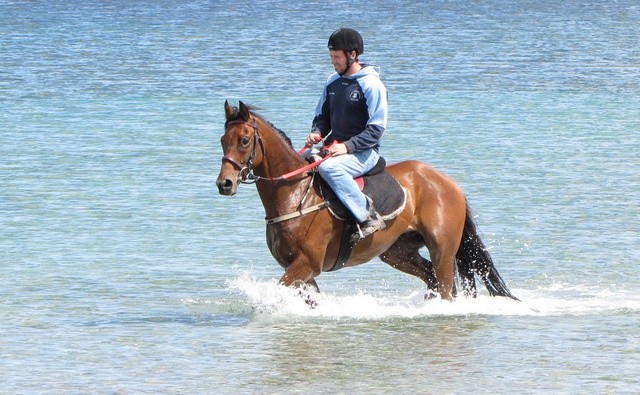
[215,99,521,308]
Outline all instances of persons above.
[303,27,389,243]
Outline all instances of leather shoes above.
[351,210,386,241]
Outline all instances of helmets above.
[328,27,363,55]
